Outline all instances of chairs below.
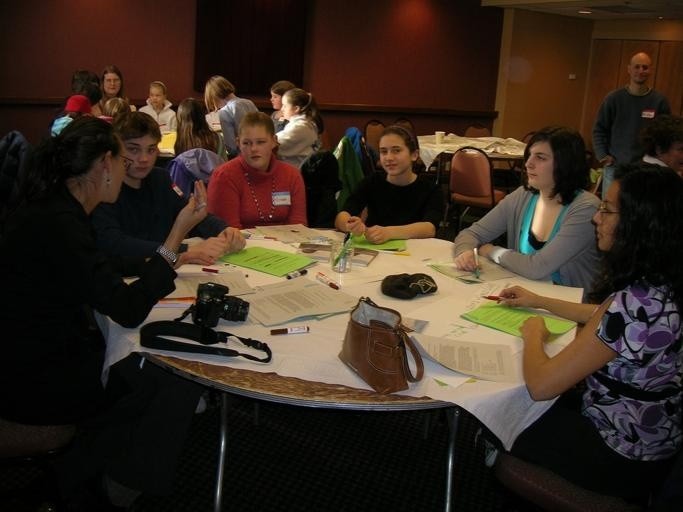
[504,131,535,188]
[465,125,491,137]
[345,125,376,173]
[481,447,683,511]
[295,150,344,227]
[330,136,365,210]
[442,146,504,240]
[1,421,78,510]
[365,121,385,149]
[173,148,225,201]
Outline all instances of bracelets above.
[156,245,180,266]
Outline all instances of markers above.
[286,269,308,279]
[338,233,351,271]
[315,271,341,290]
[270,326,310,335]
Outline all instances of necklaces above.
[244,172,276,225]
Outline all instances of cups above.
[436,131,445,144]
[331,245,351,273]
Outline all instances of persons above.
[492,163,683,511]
[0,117,210,512]
[335,120,444,244]
[206,110,308,229]
[638,113,683,178]
[592,51,672,202]
[455,124,618,303]
[91,111,247,269]
[48,65,324,197]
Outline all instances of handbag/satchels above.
[338,296,424,393]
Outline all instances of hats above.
[65,95,92,113]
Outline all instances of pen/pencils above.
[247,236,277,240]
[481,295,511,302]
[330,236,353,267]
[347,220,356,223]
[193,202,208,210]
[474,247,480,280]
[202,268,224,274]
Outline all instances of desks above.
[410,135,531,227]
[94,227,586,511]
[158,132,179,157]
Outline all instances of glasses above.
[112,154,134,170]
[597,204,619,214]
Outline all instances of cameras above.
[191,282,250,327]
